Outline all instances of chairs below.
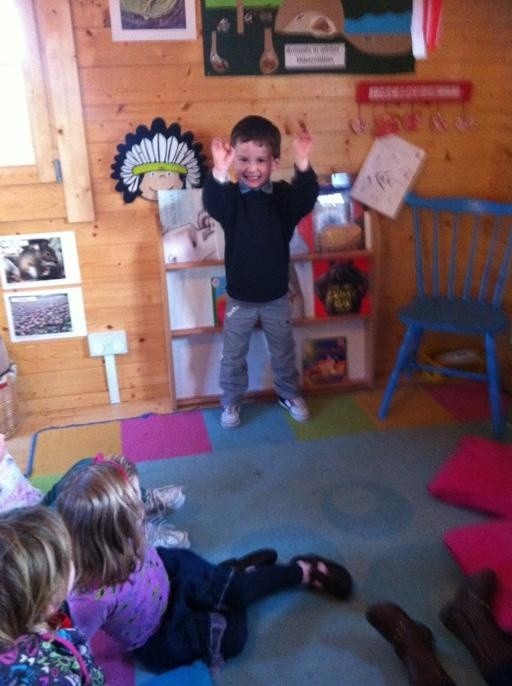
[377,193,512,436]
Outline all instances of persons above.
[364,565,511,686]
[0,431,192,557]
[44,452,354,676]
[0,505,109,686]
[203,114,319,431]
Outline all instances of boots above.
[439,566,512,685]
[364,600,455,684]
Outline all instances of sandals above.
[291,552,353,600]
[217,546,279,574]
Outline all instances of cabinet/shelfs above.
[158,209,379,410]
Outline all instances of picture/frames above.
[2,287,88,343]
[108,0,198,41]
[0,230,82,290]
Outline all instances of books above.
[209,275,260,329]
[287,192,370,256]
[310,259,372,320]
[299,336,350,385]
[350,134,427,219]
[288,264,308,323]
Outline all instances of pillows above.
[428,435,511,518]
[443,517,512,631]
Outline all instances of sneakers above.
[278,394,311,422]
[219,403,241,430]
